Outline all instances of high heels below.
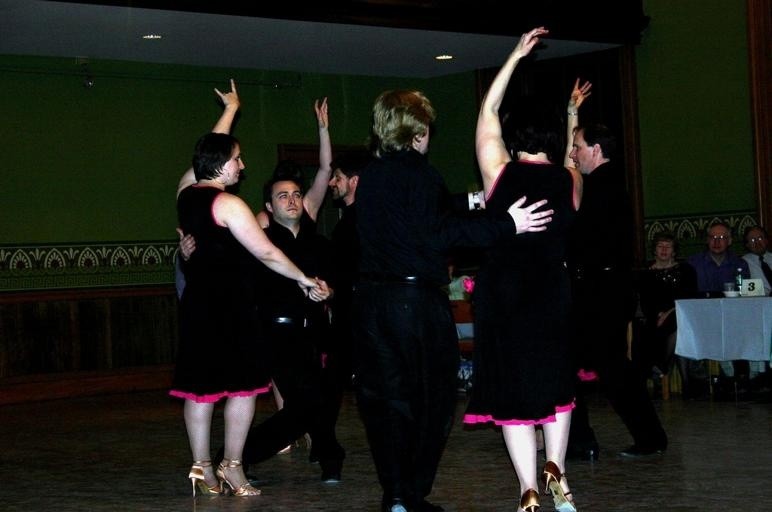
[189,460,230,498]
[216,457,262,497]
[516,489,541,512]
[541,461,577,512]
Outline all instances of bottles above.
[734,267,745,293]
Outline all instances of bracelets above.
[473,190,481,209]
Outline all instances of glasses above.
[711,235,728,241]
[750,236,764,243]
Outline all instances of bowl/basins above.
[724,291,740,298]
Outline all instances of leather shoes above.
[319,457,341,486]
[542,445,599,463]
[619,443,663,459]
[381,494,444,512]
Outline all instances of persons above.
[564,75,592,168]
[168,77,322,497]
[688,221,751,404]
[544,120,668,461]
[463,27,581,512]
[739,224,772,296]
[176,178,348,487]
[317,164,380,486]
[351,91,553,512]
[644,230,697,382]
[254,95,331,250]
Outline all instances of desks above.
[675,295,772,407]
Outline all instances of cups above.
[724,282,734,291]
